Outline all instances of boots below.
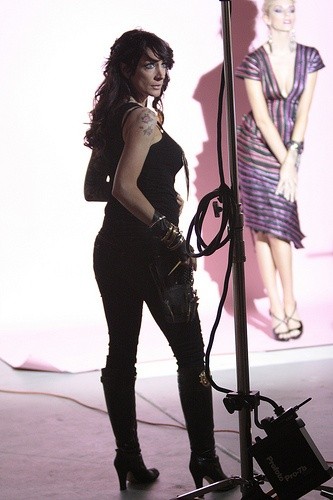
[101,368,159,490]
[177,370,236,498]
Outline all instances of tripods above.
[169,0,333,500]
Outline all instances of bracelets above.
[149,216,168,229]
[160,223,185,251]
[285,140,303,154]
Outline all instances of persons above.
[234,0,324,342]
[83,28,240,500]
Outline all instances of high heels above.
[270,308,289,341]
[285,302,302,339]
